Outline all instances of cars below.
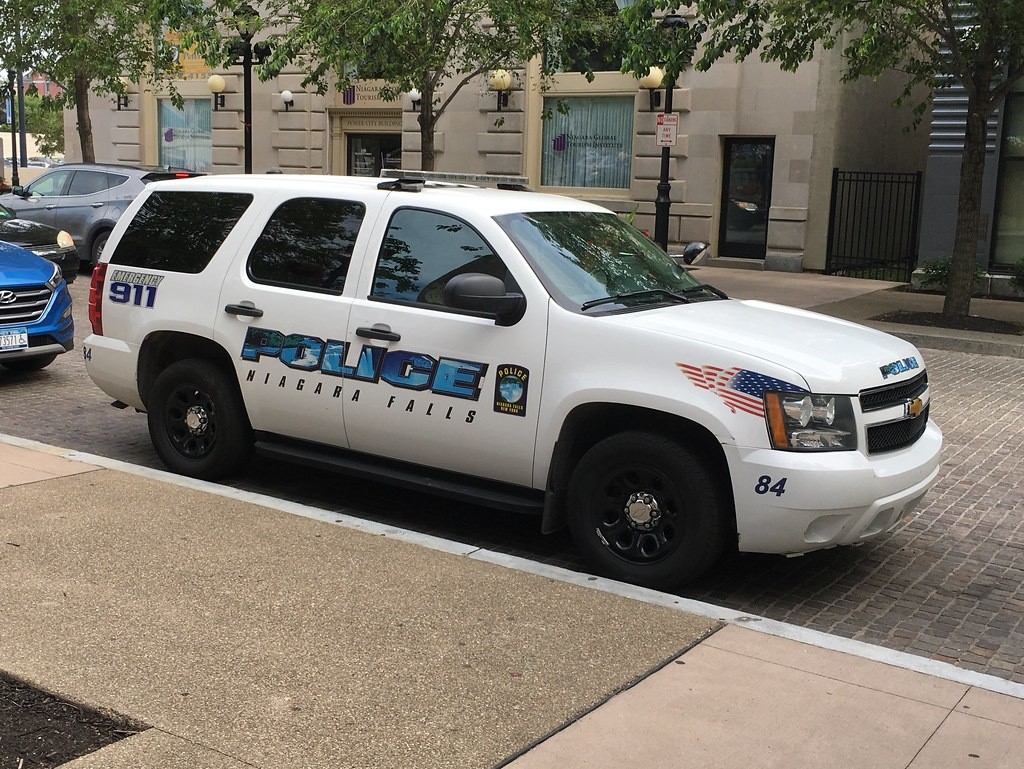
[0,241,75,372]
[0,202,81,284]
[0,204,80,284]
[0,161,211,272]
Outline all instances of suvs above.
[80,167,945,592]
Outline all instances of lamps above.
[281,90,294,112]
[640,66,664,112]
[408,88,421,111]
[208,75,225,111]
[490,69,511,111]
[111,77,128,109]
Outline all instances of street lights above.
[654,10,689,259]
[228,0,271,175]
